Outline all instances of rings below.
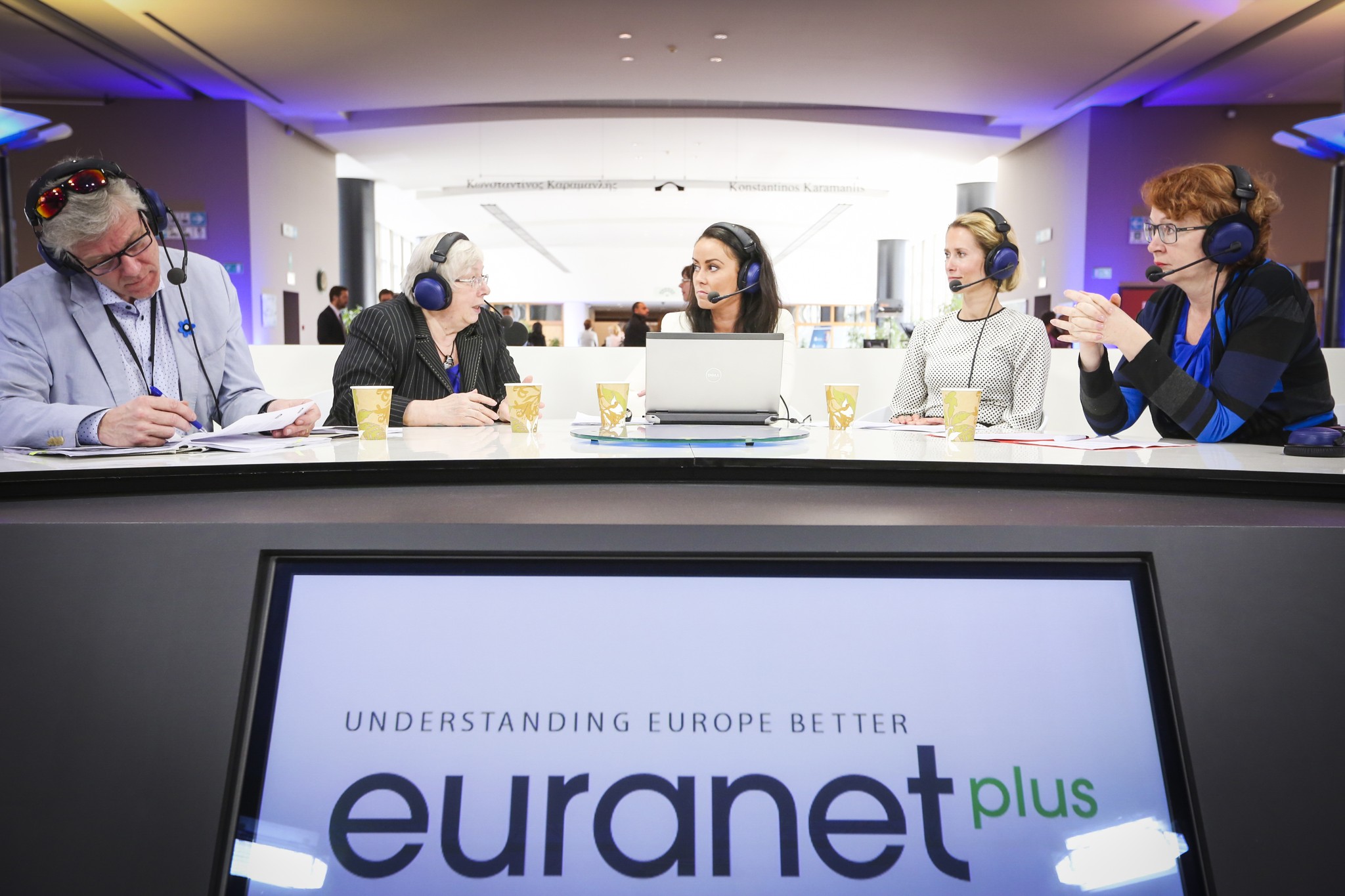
[303,428,308,436]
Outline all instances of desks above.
[0,416,1343,896]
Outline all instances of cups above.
[940,387,983,442]
[596,383,630,427]
[504,383,542,433]
[825,384,860,431]
[350,386,394,440]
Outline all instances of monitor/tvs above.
[226,553,1202,896]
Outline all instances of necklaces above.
[430,338,455,365]
[95,288,158,401]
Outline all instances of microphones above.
[1145,241,1242,283]
[949,262,1015,293]
[707,279,761,304]
[163,205,188,284]
[483,299,514,328]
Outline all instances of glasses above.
[33,173,117,221]
[682,279,691,282]
[454,275,489,288]
[65,211,153,276]
[1143,223,1209,244]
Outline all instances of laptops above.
[646,331,784,425]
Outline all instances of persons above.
[636,221,796,421]
[1050,161,1341,444]
[605,323,623,347]
[624,302,653,347]
[378,289,394,304]
[527,322,547,346]
[1037,310,1073,348]
[321,231,546,430]
[499,305,518,330]
[679,265,696,300]
[0,158,319,452]
[886,205,1052,432]
[317,285,349,345]
[578,319,600,348]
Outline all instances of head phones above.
[1201,165,1260,268]
[705,222,761,293]
[409,231,469,312]
[971,207,1020,282]
[24,156,170,277]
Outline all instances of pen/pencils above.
[149,386,210,436]
